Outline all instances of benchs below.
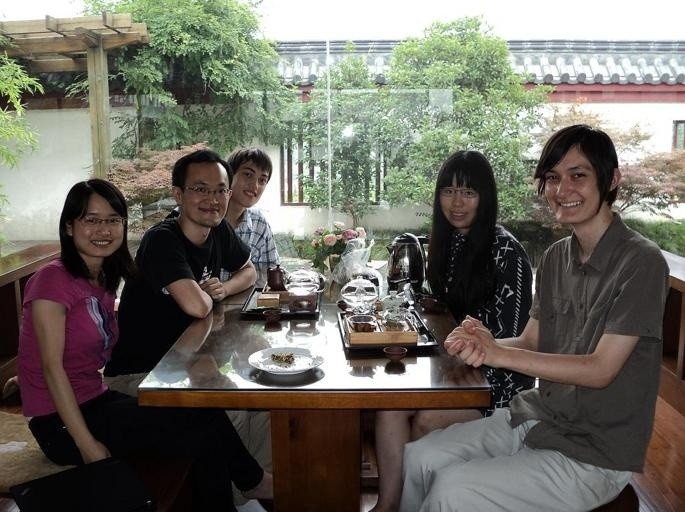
[1,243,232,512]
[516,273,685,512]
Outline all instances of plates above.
[247,347,324,376]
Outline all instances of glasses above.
[81,216,126,227]
[440,187,478,199]
[185,185,230,197]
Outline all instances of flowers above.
[310,221,371,270]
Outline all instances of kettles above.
[387,233,431,295]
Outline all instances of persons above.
[16,178,274,511]
[369,150,533,511]
[165,147,280,283]
[395,123,670,511]
[103,149,271,511]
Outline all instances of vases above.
[325,255,341,302]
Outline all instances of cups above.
[384,346,408,363]
[348,315,377,332]
[418,298,438,311]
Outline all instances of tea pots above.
[340,276,378,308]
[266,264,286,289]
[263,309,281,324]
[381,290,414,331]
[283,267,328,295]
